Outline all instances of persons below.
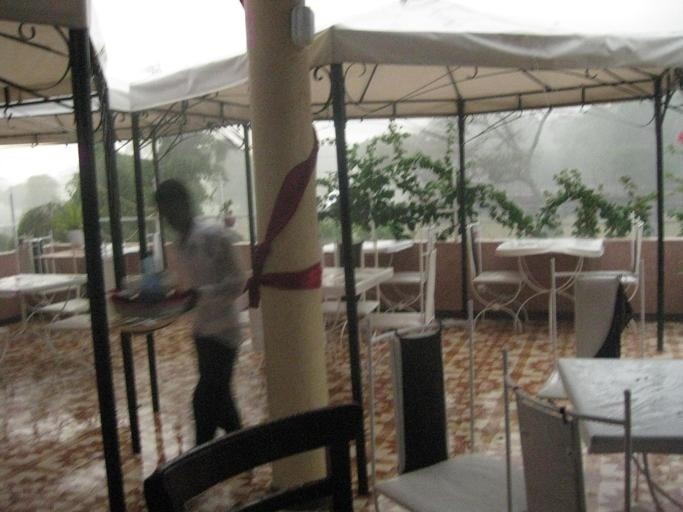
[126,176,249,452]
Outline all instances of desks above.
[556,357,682,512]
[492,235,608,352]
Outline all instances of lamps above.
[290,4,315,47]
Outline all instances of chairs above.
[0,228,162,354]
[558,218,648,351]
[373,348,638,510]
[144,404,370,512]
[461,221,529,335]
[323,217,447,366]
